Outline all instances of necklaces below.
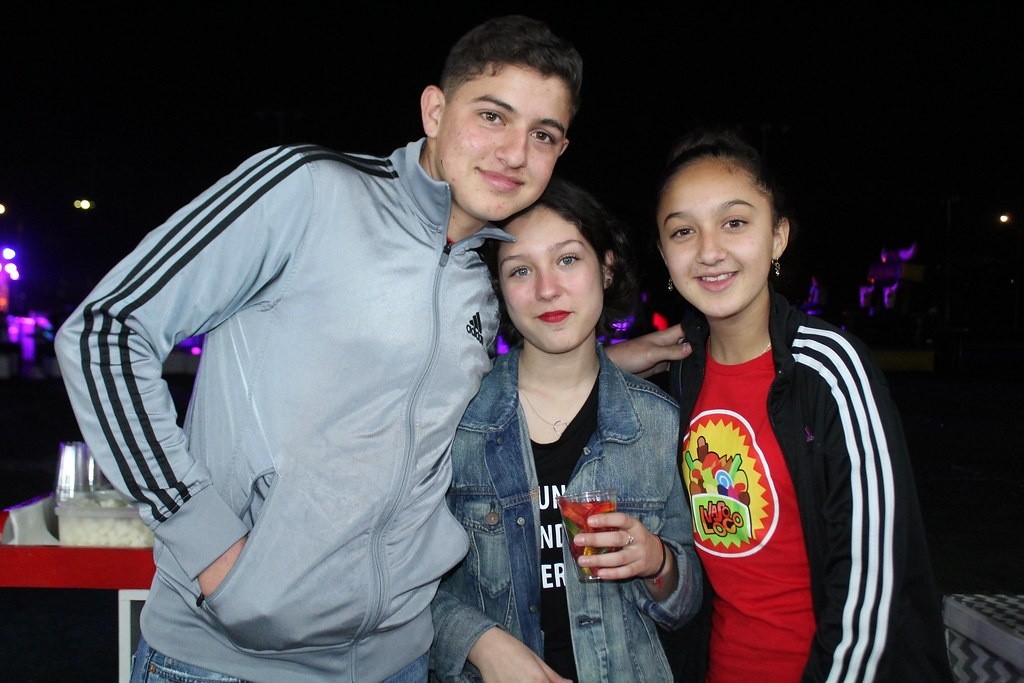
[762,343,771,354]
[519,386,567,434]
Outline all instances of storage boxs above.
[55,491,156,549]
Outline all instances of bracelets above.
[644,534,666,582]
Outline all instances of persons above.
[54,14,692,683]
[428,178,704,683]
[655,129,953,683]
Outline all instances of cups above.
[556,488,619,580]
[53,442,100,504]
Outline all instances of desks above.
[0,495,156,683]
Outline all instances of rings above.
[625,531,634,546]
